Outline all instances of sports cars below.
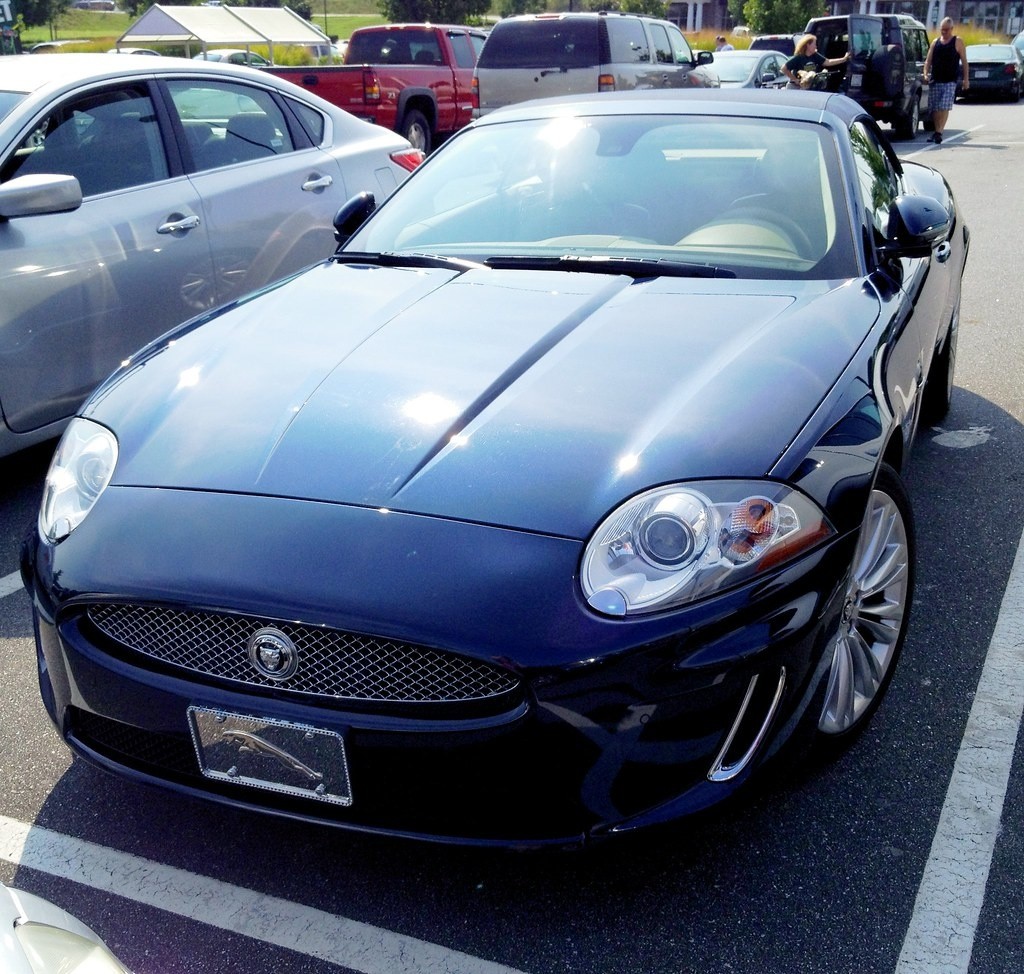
[15,78,973,850]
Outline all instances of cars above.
[696,50,794,89]
[956,43,1024,102]
[1010,29,1024,60]
[72,0,115,11]
[192,48,279,68]
[0,50,440,457]
[30,39,94,54]
[331,39,350,63]
[106,47,162,57]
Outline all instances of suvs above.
[469,9,719,125]
[749,30,802,58]
[804,11,931,139]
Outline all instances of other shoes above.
[933,135,942,144]
[926,133,934,143]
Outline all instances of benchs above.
[381,47,445,63]
[182,112,280,168]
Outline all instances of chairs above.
[67,117,168,195]
[735,137,828,263]
[533,140,670,243]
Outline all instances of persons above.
[923,16,971,143]
[780,34,851,90]
[714,36,722,52]
[127,7,135,22]
[719,36,734,51]
[329,35,345,58]
[13,112,97,215]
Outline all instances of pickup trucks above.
[257,23,490,157]
